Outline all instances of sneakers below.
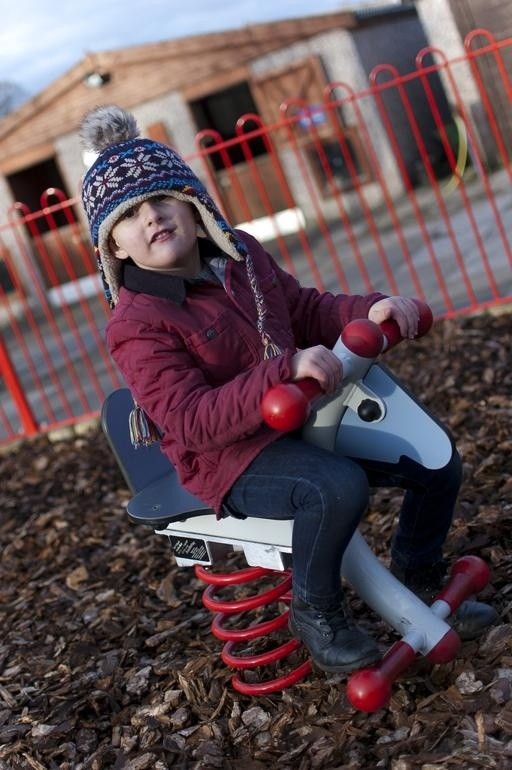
[390,561,500,640]
[287,595,380,674]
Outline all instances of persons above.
[80,104,503,675]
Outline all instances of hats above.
[80,105,244,309]
